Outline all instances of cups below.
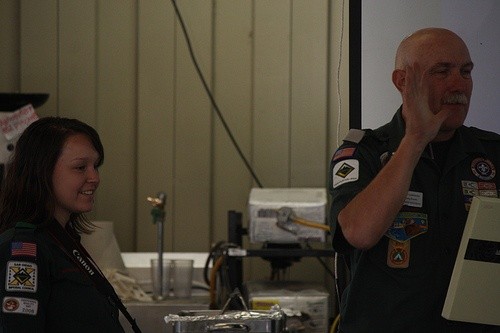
[151,259,172,299]
[172,259,194,299]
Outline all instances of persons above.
[0,116,127,333]
[329,27,500,333]
